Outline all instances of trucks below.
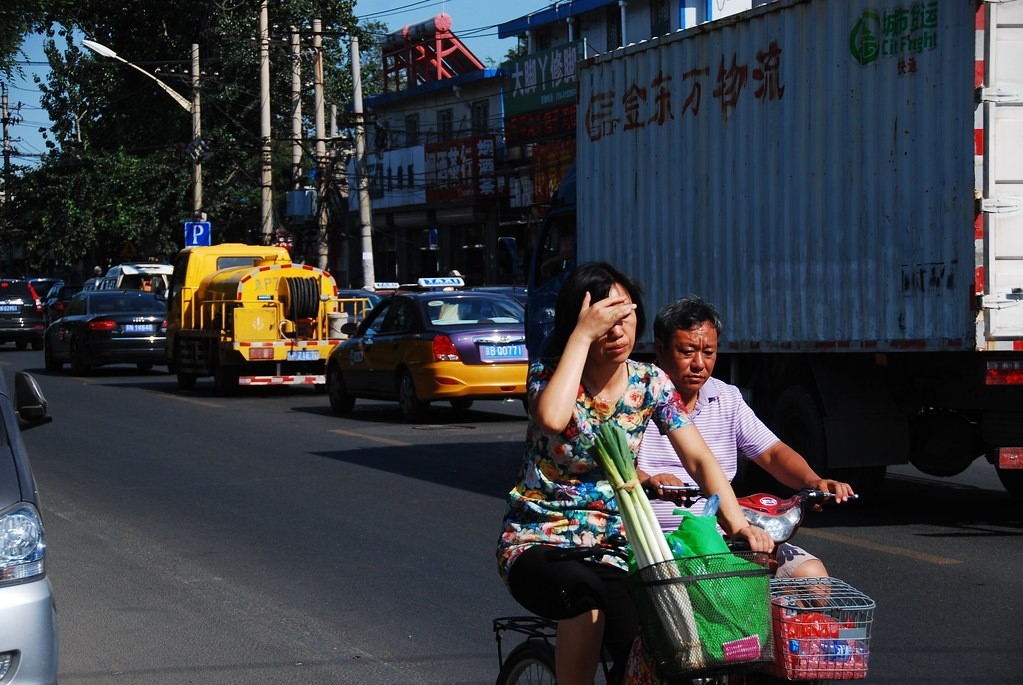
[534,0,1023,512]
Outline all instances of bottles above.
[789,640,869,664]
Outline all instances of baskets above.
[628,550,777,681]
[768,577,876,680]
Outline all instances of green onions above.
[578,419,705,670]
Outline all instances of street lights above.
[80,37,205,222]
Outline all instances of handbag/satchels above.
[625,508,769,662]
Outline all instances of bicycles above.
[493,539,779,685]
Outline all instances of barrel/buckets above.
[327,312,349,338]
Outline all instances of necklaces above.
[584,367,626,402]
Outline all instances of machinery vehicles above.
[149,242,370,397]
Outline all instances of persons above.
[635,295,854,610]
[493,262,774,685]
[560,234,575,257]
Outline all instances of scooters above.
[642,483,877,685]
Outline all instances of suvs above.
[0,261,174,351]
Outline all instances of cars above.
[324,275,528,424]
[0,365,60,685]
[336,282,530,336]
[41,290,168,378]
